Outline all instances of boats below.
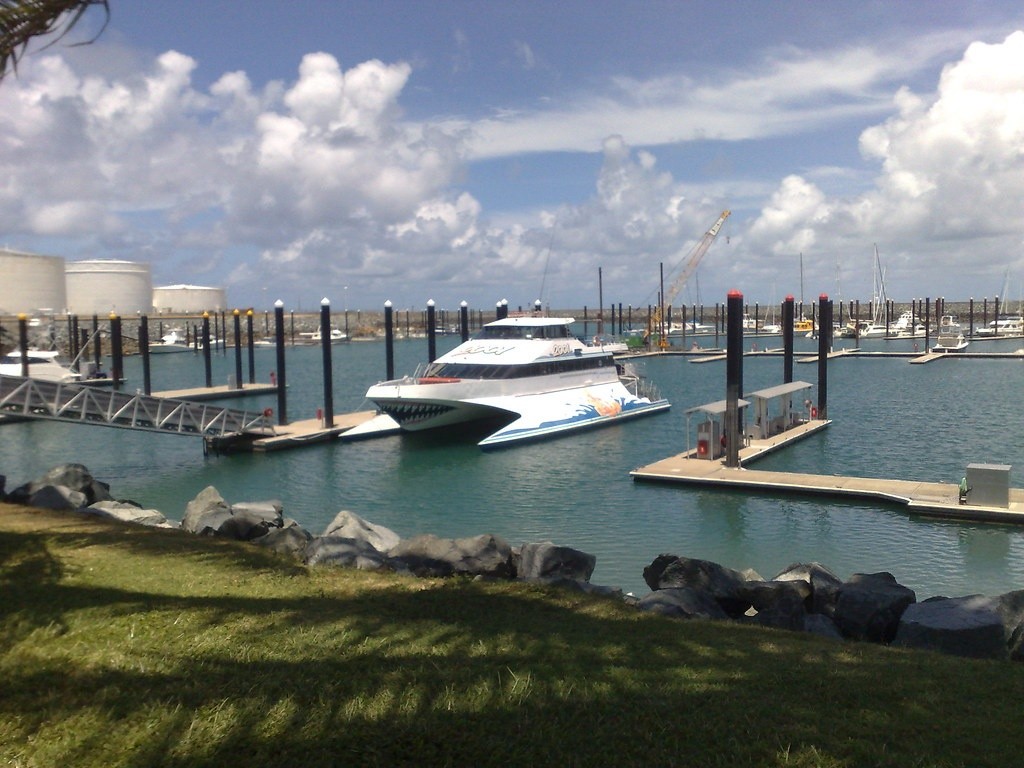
[298,322,353,345]
[149,339,194,353]
[0,315,109,383]
[188,334,227,350]
[253,340,277,348]
[336,316,671,450]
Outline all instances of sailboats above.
[621,241,1024,339]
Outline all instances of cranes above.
[621,208,734,350]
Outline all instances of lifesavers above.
[593,335,600,347]
